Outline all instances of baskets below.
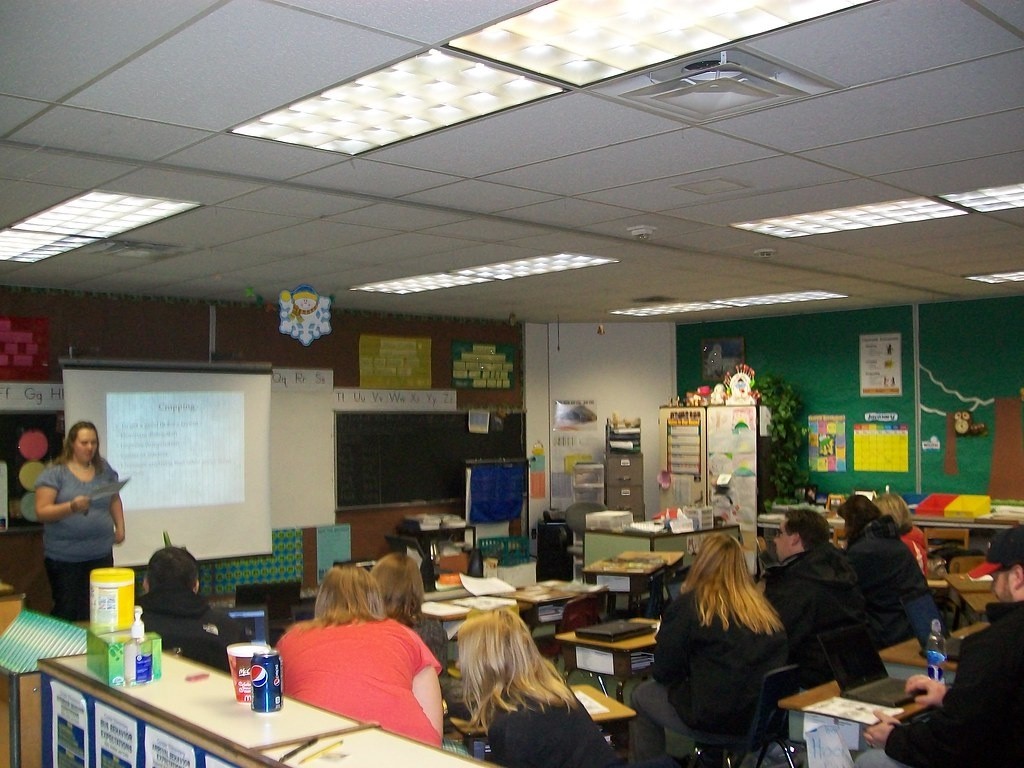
[480,537,529,566]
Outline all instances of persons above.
[37,422,126,622]
[135,547,257,679]
[456,607,626,768]
[272,553,448,750]
[627,491,1024,767]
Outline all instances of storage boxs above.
[478,537,538,588]
[86,626,162,688]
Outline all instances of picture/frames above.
[700,336,745,381]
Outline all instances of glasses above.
[775,528,791,538]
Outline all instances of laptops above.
[818,624,929,707]
[217,605,270,646]
[574,621,657,642]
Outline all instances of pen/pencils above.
[278,738,318,766]
[300,740,344,766]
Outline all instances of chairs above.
[568,502,990,767]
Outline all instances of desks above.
[422,573,1004,768]
[397,525,474,572]
[581,524,743,572]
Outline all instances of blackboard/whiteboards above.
[334,409,527,512]
[2,408,67,536]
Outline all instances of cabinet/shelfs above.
[603,418,646,521]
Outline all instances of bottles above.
[925,619,948,686]
[429,541,441,579]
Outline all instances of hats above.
[969,526,1023,578]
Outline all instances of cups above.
[227,642,272,703]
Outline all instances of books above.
[404,513,466,532]
